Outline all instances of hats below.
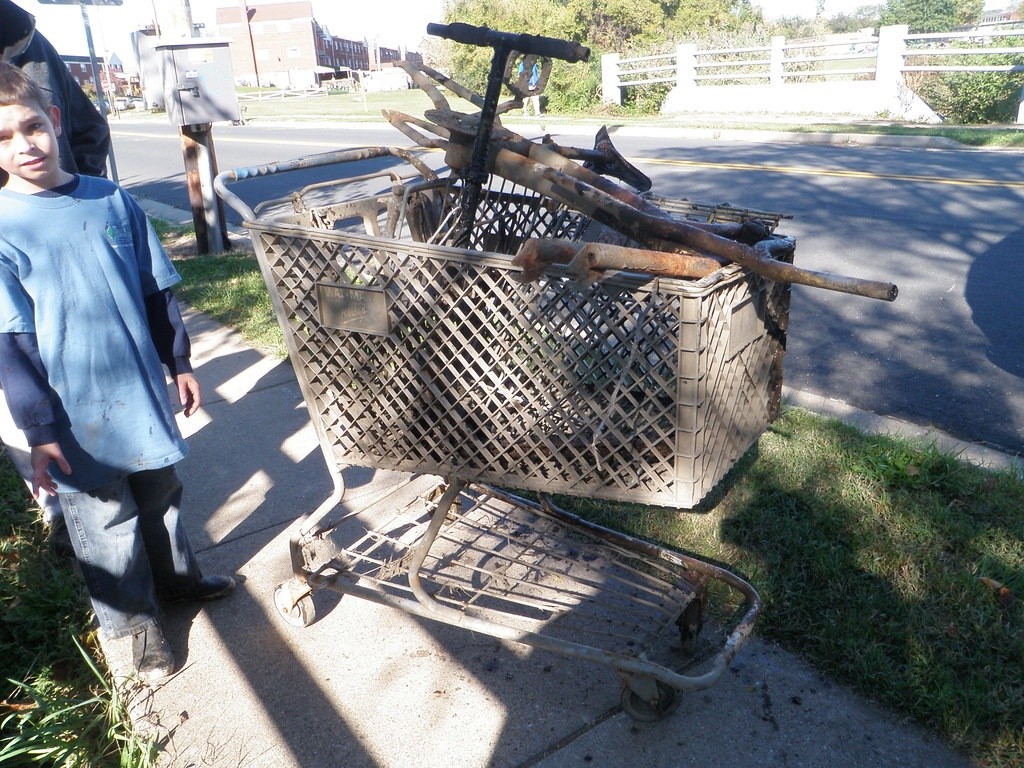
[0,0,35,61]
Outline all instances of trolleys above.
[213,140,798,720]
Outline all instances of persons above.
[0,0,112,545]
[0,62,237,679]
[519,60,546,118]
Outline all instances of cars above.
[92,96,130,114]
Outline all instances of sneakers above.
[132,624,174,679]
[190,575,236,599]
